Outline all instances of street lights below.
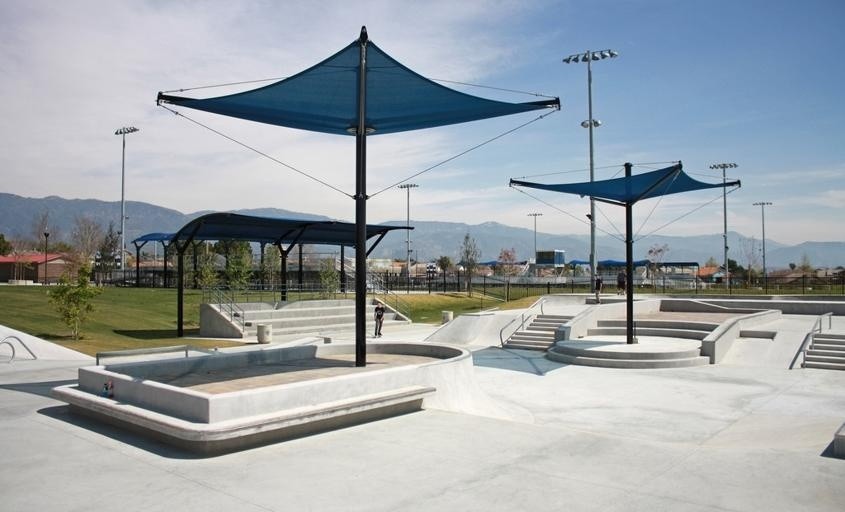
[564,49,618,293]
[114,124,139,269]
[754,202,772,276]
[44,232,49,284]
[710,162,738,290]
[398,183,419,279]
[527,212,543,252]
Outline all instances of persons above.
[595,274,602,303]
[617,269,627,295]
[372,301,386,335]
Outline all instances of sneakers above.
[375,332,381,336]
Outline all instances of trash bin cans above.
[257,324,273,344]
[442,310,453,324]
